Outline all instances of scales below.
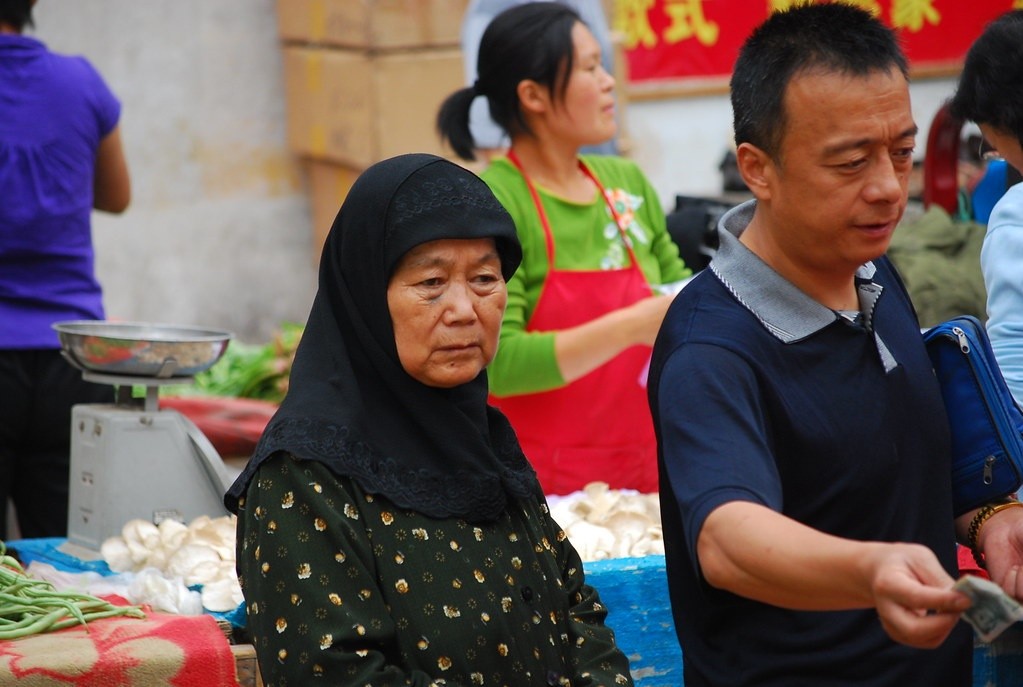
[50,319,237,564]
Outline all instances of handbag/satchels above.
[922,315,1023,519]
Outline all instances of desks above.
[0,380,687,687]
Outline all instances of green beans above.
[0,541,146,641]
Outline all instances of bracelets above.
[967,498,1023,572]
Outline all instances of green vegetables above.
[131,320,306,403]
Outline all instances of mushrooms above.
[544,480,666,562]
[101,512,243,613]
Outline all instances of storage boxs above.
[278,0,468,267]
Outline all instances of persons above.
[643,0,1023,687]
[222,154,634,687]
[0,0,132,541]
[433,1,697,498]
[953,6,1023,509]
